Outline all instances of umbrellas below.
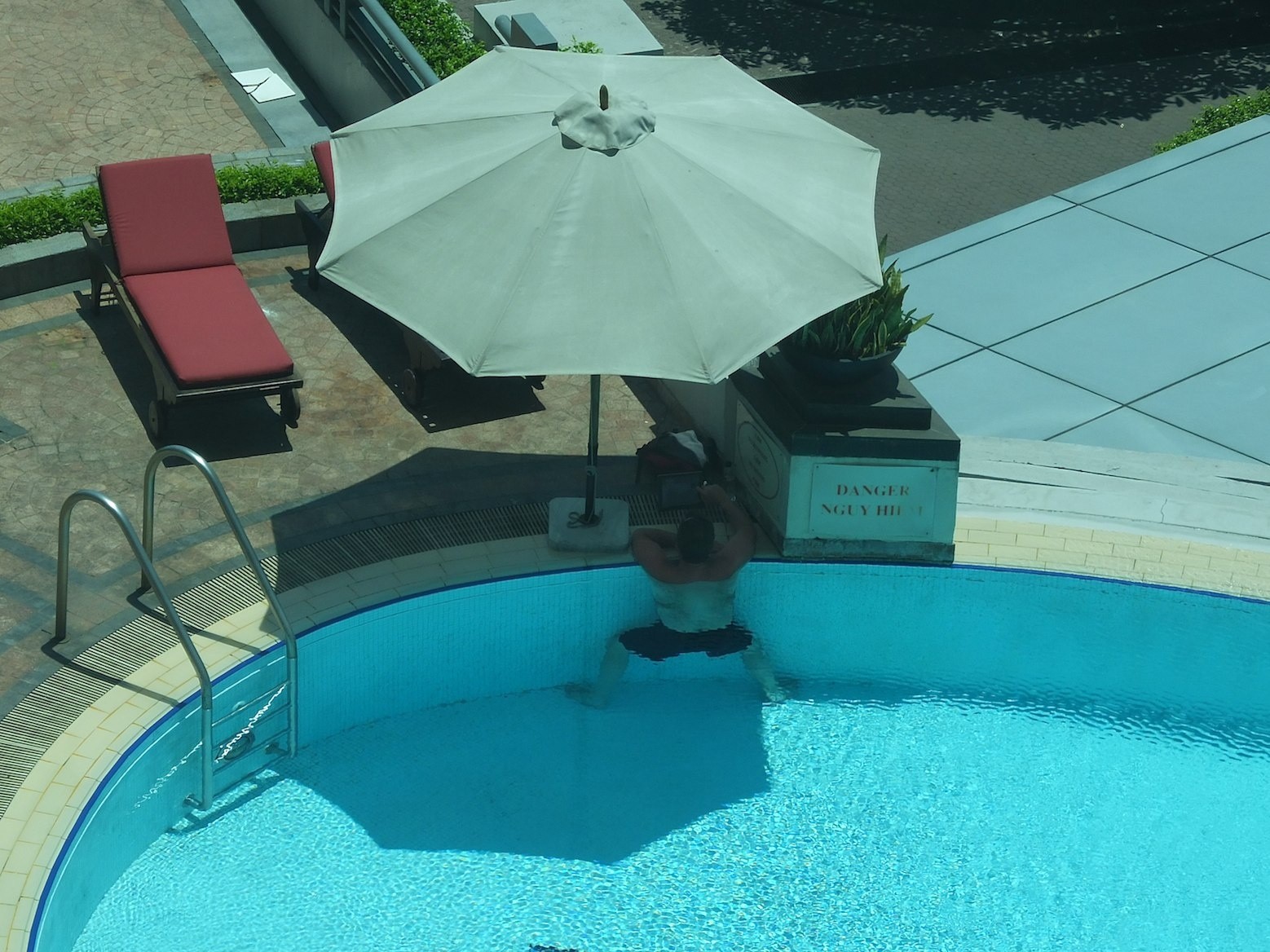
[313,44,888,531]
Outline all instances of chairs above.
[294,140,547,404]
[82,153,304,436]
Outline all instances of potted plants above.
[777,233,935,387]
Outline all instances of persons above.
[564,482,799,712]
